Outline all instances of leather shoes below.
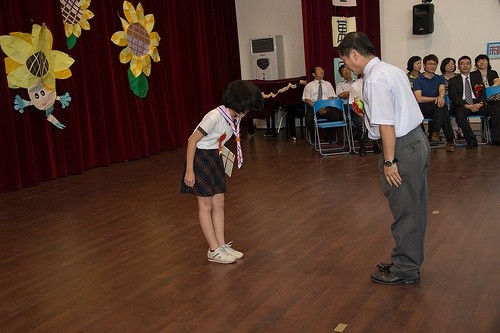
[369,262,420,285]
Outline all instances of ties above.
[317,80,323,101]
[465,77,473,105]
[360,73,367,139]
[217,105,244,170]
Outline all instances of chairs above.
[304,85,500,157]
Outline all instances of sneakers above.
[207,241,244,264]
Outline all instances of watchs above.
[384,158,398,166]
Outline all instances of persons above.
[405,54,456,153]
[440,54,500,158]
[302,65,343,146]
[339,32,430,284]
[336,65,382,157]
[179,80,262,264]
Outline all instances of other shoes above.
[466,142,478,149]
[446,143,456,152]
[359,140,367,157]
[373,143,381,154]
[430,132,439,142]
[491,139,500,145]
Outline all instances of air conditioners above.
[249,34,287,128]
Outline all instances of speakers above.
[412,4,435,34]
